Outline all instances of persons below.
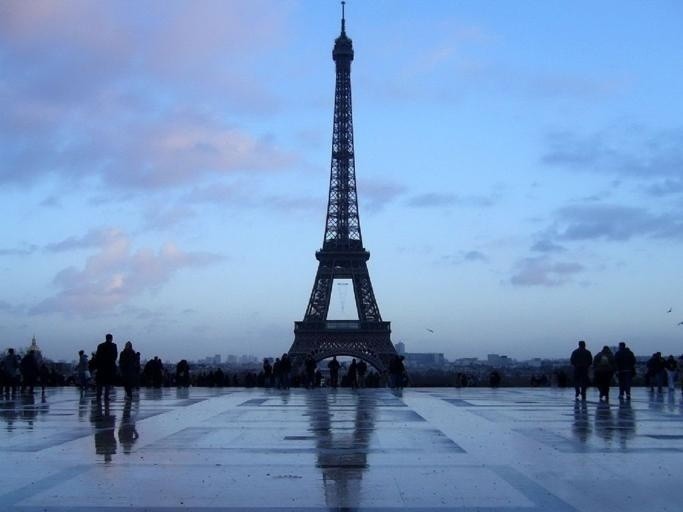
[196,353,407,390]
[1,335,191,401]
[572,341,676,399]
